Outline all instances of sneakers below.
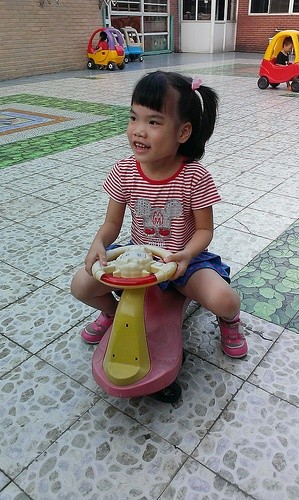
[215,312,248,358]
[80,301,120,345]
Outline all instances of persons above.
[93,31,108,69]
[70,70,248,359]
[124,34,134,44]
[274,36,294,91]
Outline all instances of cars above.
[87,28,126,71]
[117,26,144,64]
[257,30,299,93]
